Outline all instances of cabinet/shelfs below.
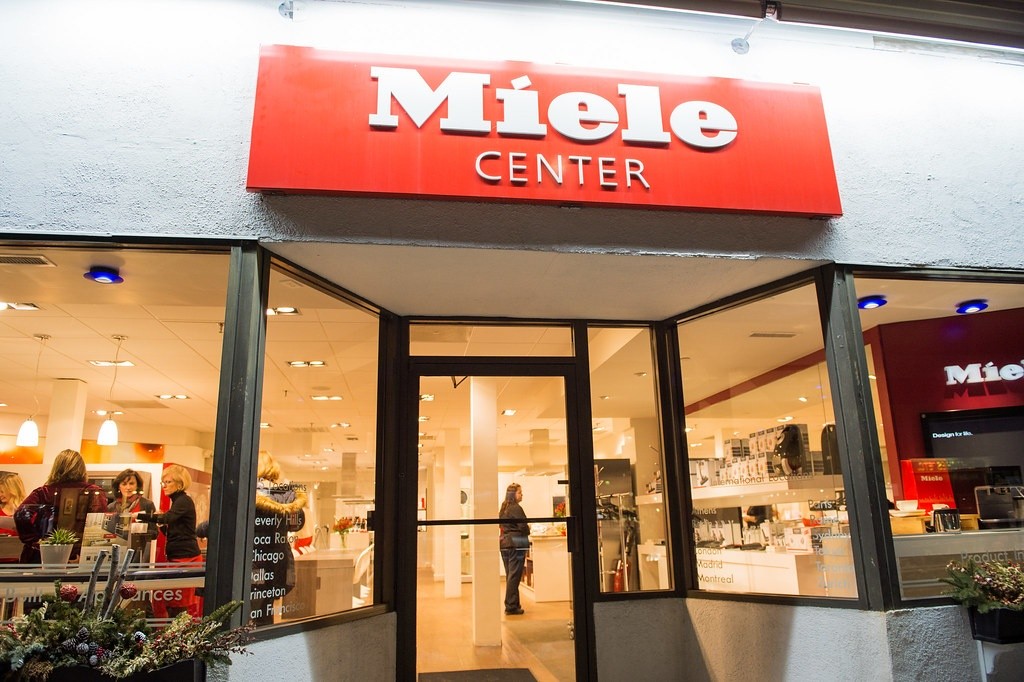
[634,473,843,596]
[517,536,573,601]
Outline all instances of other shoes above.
[504,606,525,615]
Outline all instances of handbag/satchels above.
[509,534,530,551]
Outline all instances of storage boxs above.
[809,487,850,523]
[743,521,830,551]
[690,424,813,492]
[645,470,661,494]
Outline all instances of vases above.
[340,532,348,549]
[561,531,566,536]
[967,605,1024,644]
[0,656,207,682]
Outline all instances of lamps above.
[956,300,988,313]
[16,334,52,447]
[277,0,305,21]
[858,295,886,309]
[732,17,778,55]
[84,266,125,284]
[97,335,129,447]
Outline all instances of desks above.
[0,567,205,624]
[280,547,370,619]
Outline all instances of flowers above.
[936,557,1024,615]
[333,515,354,534]
[0,578,256,679]
[555,501,566,533]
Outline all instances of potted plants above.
[40,525,80,570]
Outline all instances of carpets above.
[418,668,538,682]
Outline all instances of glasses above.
[160,479,178,486]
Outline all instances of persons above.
[743,504,773,529]
[196,450,307,626]
[132,465,203,624]
[0,472,36,566]
[107,469,159,580]
[14,449,107,618]
[498,483,530,614]
